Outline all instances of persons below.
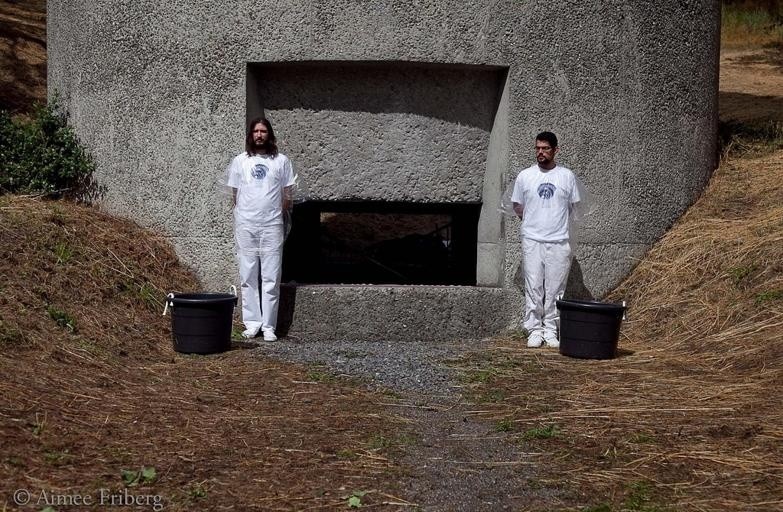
[226,116,295,343]
[511,131,581,349]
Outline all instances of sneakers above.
[528,334,543,348]
[241,330,255,339]
[263,331,277,342]
[545,337,560,348]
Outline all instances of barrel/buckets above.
[162,284,237,356]
[554,291,627,359]
